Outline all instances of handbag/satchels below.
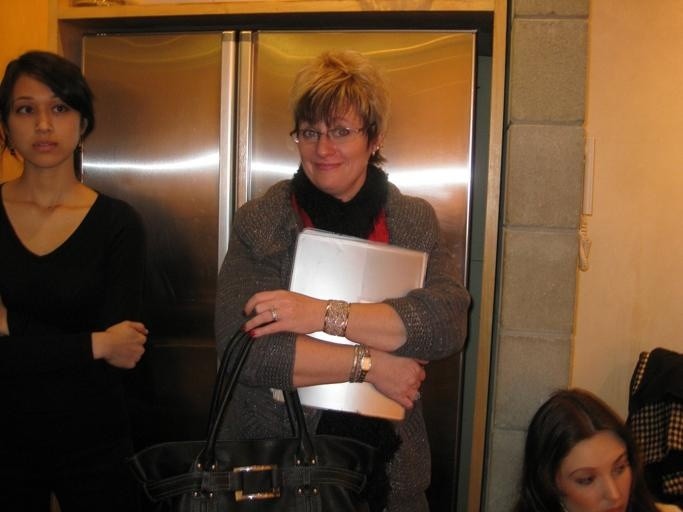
[125,433,377,512]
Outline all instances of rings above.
[269,308,277,321]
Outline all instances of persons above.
[0,49,150,512]
[205,46,471,511]
[511,386,682,512]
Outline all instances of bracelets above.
[348,344,363,383]
[323,299,352,337]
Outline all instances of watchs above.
[358,343,371,384]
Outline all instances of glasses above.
[290,124,376,142]
